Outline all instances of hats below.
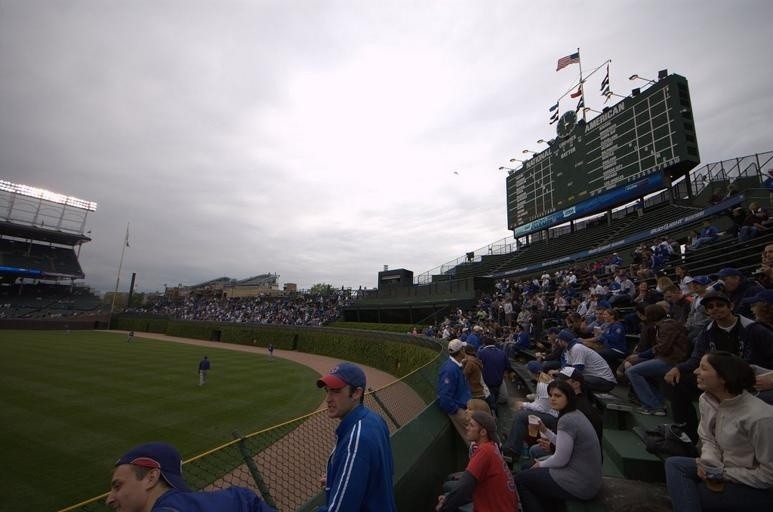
[114,443,193,493]
[316,363,366,388]
[471,412,499,444]
[447,339,468,354]
[682,268,772,306]
[548,299,611,343]
[429,325,484,335]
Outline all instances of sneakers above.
[640,408,666,416]
[526,394,536,400]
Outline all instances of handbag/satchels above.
[645,421,700,462]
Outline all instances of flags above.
[549,74,609,125]
[556,52,579,71]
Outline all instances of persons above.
[128,328,134,343]
[268,343,273,362]
[198,355,210,386]
[124,291,350,325]
[316,363,398,512]
[409,169,772,512]
[106,442,278,512]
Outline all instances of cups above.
[540,430,549,442]
[593,327,601,337]
[528,415,540,437]
[705,280,726,293]
[699,459,725,491]
